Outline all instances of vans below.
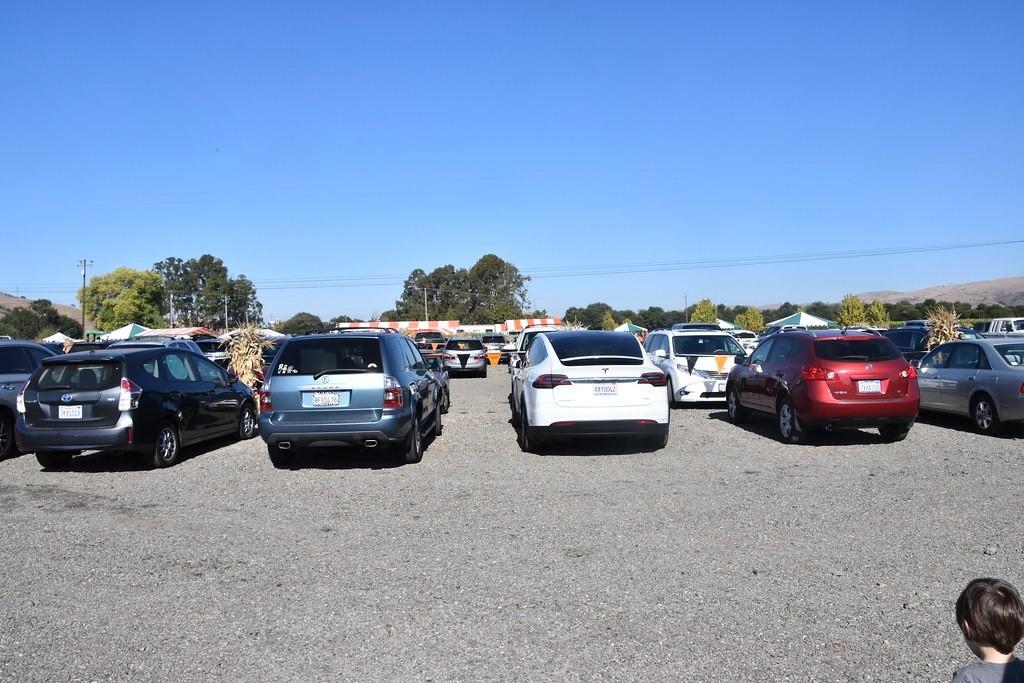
[672,322,721,330]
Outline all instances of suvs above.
[642,328,747,410]
[258,326,444,465]
[726,325,922,444]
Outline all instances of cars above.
[510,330,670,451]
[226,334,289,388]
[439,335,488,378]
[722,324,809,360]
[909,334,1024,435]
[835,316,1024,361]
[415,325,558,364]
[0,334,229,464]
[12,347,260,469]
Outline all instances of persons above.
[413,334,429,349]
[949,576,1024,683]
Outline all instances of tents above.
[714,317,742,330]
[493,317,563,335]
[335,319,461,339]
[613,322,649,340]
[218,325,288,342]
[36,321,218,345]
[765,310,839,335]
